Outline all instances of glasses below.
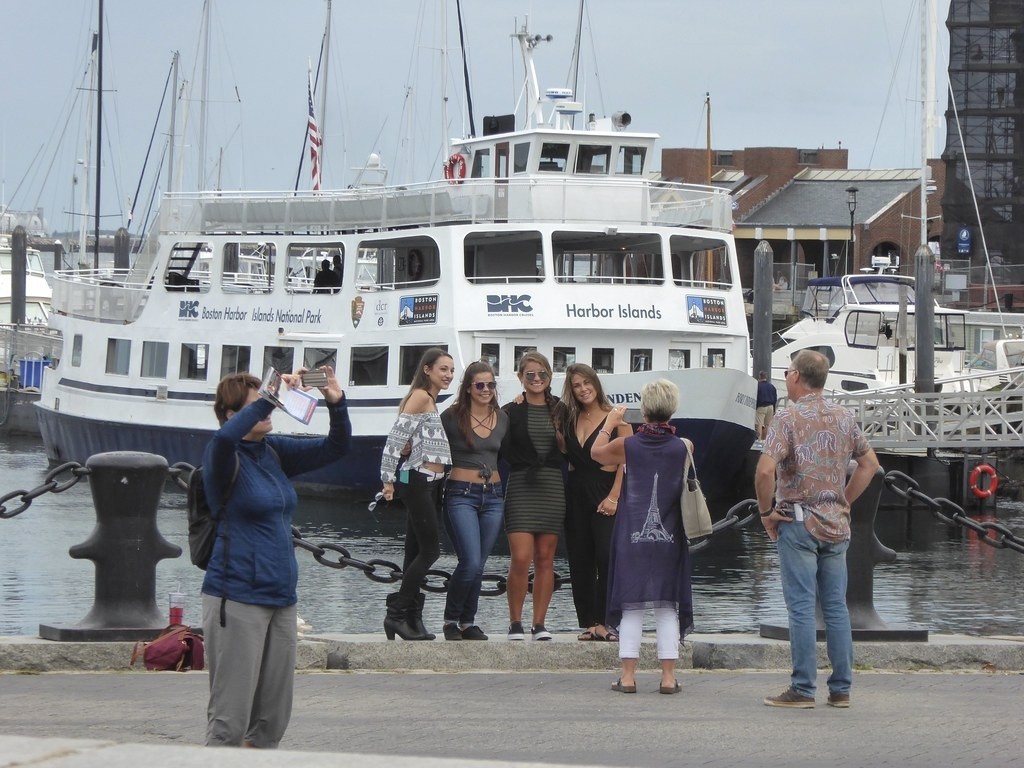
[785,370,796,376]
[522,371,548,380]
[368,492,383,511]
[471,381,497,389]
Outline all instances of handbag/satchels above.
[130,623,204,671]
[680,438,713,539]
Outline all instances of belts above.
[414,467,445,482]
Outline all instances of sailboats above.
[1,13,227,439]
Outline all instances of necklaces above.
[585,410,589,415]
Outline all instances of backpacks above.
[187,443,280,571]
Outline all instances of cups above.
[168,593,186,625]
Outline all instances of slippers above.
[660,678,682,693]
[595,628,618,642]
[578,631,597,640]
[611,677,636,692]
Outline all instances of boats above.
[745,1,1024,510]
[31,0,762,501]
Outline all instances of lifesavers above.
[969,465,999,498]
[445,153,466,185]
[406,250,423,281]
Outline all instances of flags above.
[305,78,324,196]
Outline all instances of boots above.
[410,593,436,640]
[384,592,425,640]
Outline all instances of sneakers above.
[531,625,552,640]
[508,621,524,640]
[462,626,488,640]
[828,693,850,707]
[443,623,462,639]
[764,686,815,708]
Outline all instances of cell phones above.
[302,370,329,387]
[623,409,645,424]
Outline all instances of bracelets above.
[601,429,611,437]
[761,507,773,517]
[607,496,618,504]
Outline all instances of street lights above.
[846,184,859,274]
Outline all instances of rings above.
[615,408,617,410]
[601,509,605,513]
[605,511,608,515]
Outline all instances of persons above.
[590,377,697,693]
[313,260,340,294]
[756,370,777,440]
[753,350,877,709]
[378,347,454,640]
[332,255,343,278]
[499,351,571,642]
[399,357,512,639]
[773,275,788,290]
[511,363,626,645]
[199,364,353,751]
